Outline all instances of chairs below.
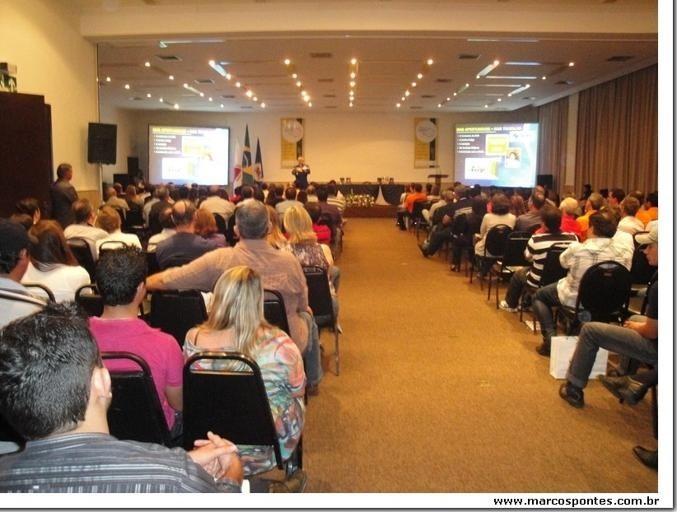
[0,283,55,328]
[96,350,171,445]
[446,215,658,403]
[409,199,432,241]
[181,350,304,471]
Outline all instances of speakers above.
[537,174,554,190]
[127,157,138,177]
[87,122,117,164]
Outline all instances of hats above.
[633,224,658,246]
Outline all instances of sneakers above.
[270,469,308,492]
[497,299,519,313]
[533,342,550,356]
[415,239,434,258]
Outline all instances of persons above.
[292,156,310,189]
[88,244,185,449]
[182,265,307,477]
[396,181,658,469]
[49,164,78,219]
[0,179,347,394]
[0,300,308,493]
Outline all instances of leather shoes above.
[630,445,657,471]
[597,371,648,407]
[450,263,460,272]
[557,382,585,408]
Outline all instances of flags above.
[233,123,264,196]
